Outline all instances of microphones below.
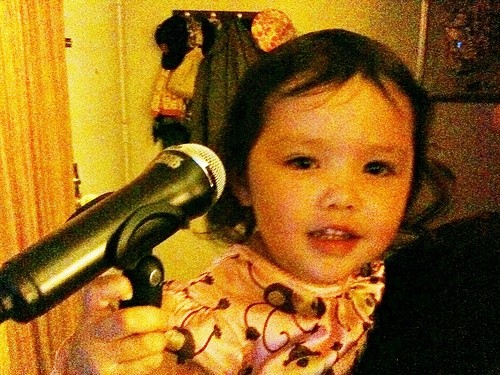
[0,142,229,327]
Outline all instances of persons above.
[44,26,454,374]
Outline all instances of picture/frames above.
[410,0,499,104]
